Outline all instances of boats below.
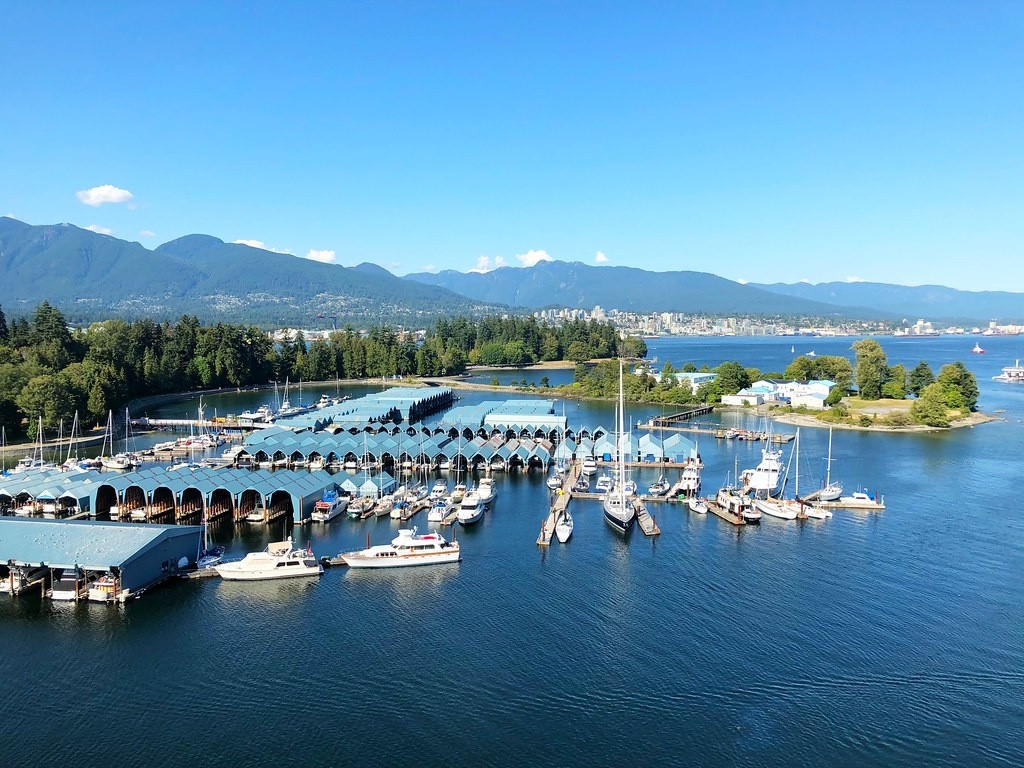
[715,456,763,526]
[46,569,97,601]
[206,530,327,580]
[0,565,51,597]
[338,525,461,568]
[88,572,121,604]
[838,482,885,506]
[688,495,709,514]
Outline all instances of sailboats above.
[0,373,500,526]
[194,493,227,570]
[816,424,844,502]
[547,413,703,498]
[600,361,637,537]
[716,409,834,521]
[553,401,574,544]
